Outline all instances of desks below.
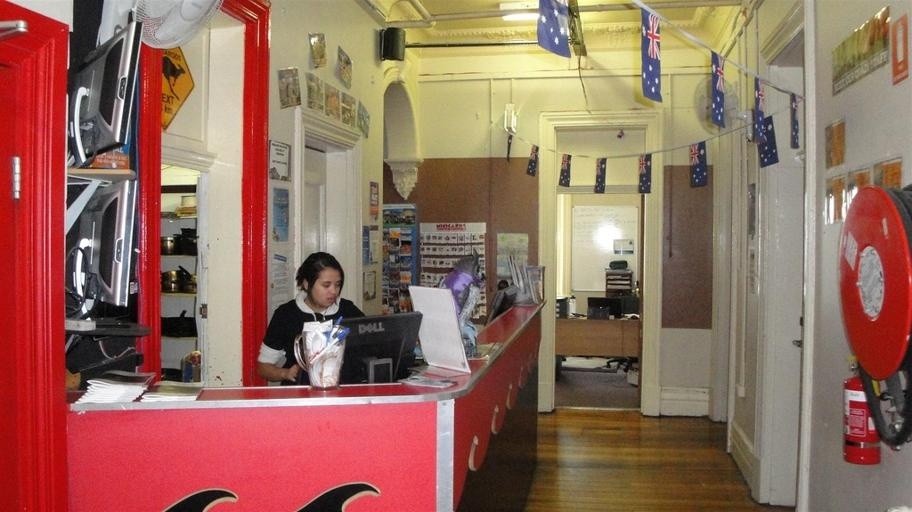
[554,318,640,380]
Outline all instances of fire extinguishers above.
[840,362,881,466]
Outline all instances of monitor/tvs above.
[67,180,138,329]
[298,311,422,384]
[67,21,143,169]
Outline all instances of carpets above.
[554,367,638,410]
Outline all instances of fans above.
[131,1,224,50]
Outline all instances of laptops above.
[587,297,623,318]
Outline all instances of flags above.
[637,152,653,193]
[688,140,706,187]
[711,51,727,128]
[755,117,780,168]
[537,1,572,58]
[558,154,572,188]
[791,95,800,150]
[525,145,540,178]
[506,135,513,163]
[640,7,664,104]
[595,157,607,193]
[754,78,765,144]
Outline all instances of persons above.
[257,252,367,386]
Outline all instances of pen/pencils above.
[307,314,350,365]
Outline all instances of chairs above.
[607,295,639,373]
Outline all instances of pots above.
[158,227,197,336]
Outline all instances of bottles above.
[568,294,576,317]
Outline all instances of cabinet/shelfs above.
[606,270,633,297]
[160,184,197,339]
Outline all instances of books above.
[397,374,457,389]
[604,266,633,290]
[73,369,206,405]
[508,253,545,309]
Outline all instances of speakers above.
[379,27,406,61]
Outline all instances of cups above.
[293,325,349,393]
